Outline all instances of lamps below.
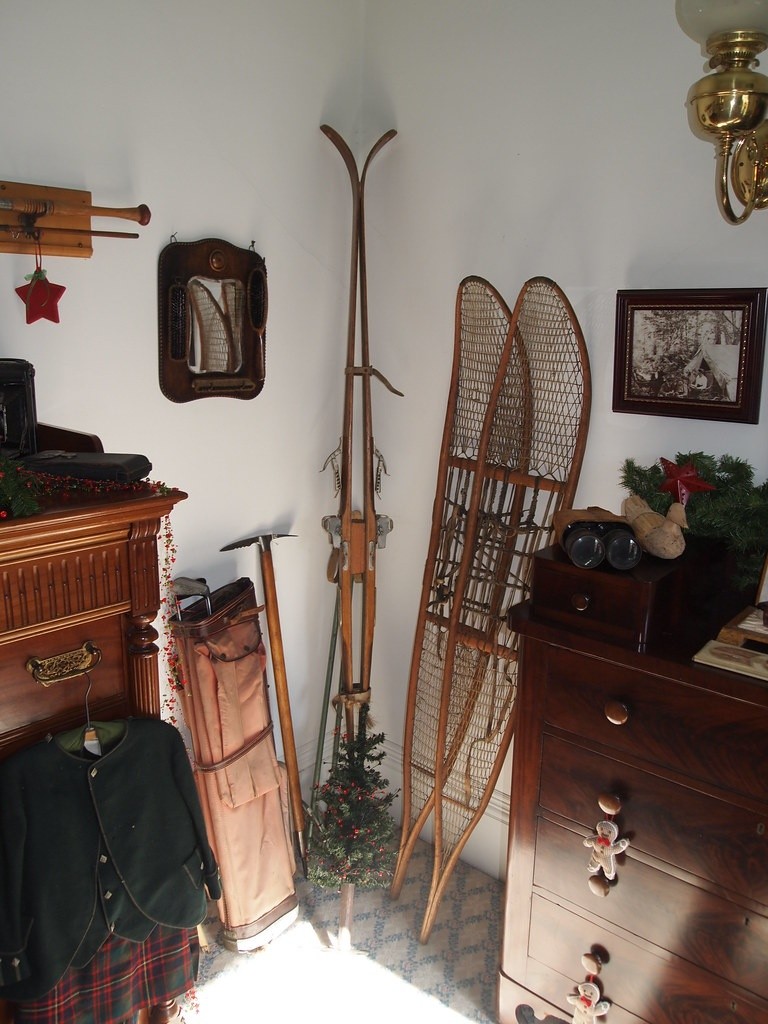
[674,0,768,227]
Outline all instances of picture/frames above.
[609,288,766,426]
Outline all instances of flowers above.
[0,463,200,785]
[304,701,403,893]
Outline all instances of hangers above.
[55,668,126,753]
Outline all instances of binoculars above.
[564,524,643,570]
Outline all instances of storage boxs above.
[529,534,733,652]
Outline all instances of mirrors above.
[157,237,268,403]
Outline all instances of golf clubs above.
[170,576,212,622]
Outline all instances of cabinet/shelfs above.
[1,478,189,1023]
[494,599,768,1023]
